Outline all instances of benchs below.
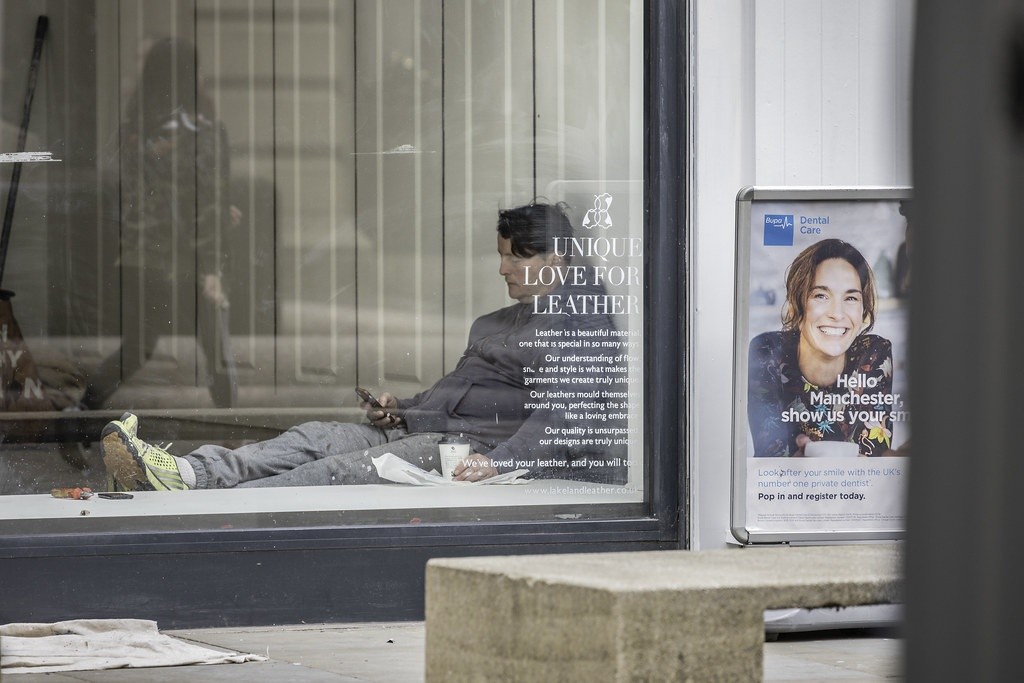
[424,538,903,683]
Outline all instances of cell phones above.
[355,387,395,424]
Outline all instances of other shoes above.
[58,407,93,474]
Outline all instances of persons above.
[747,238,896,457]
[59,34,244,472]
[99,202,619,494]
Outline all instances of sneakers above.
[106,411,138,491]
[100,421,190,491]
